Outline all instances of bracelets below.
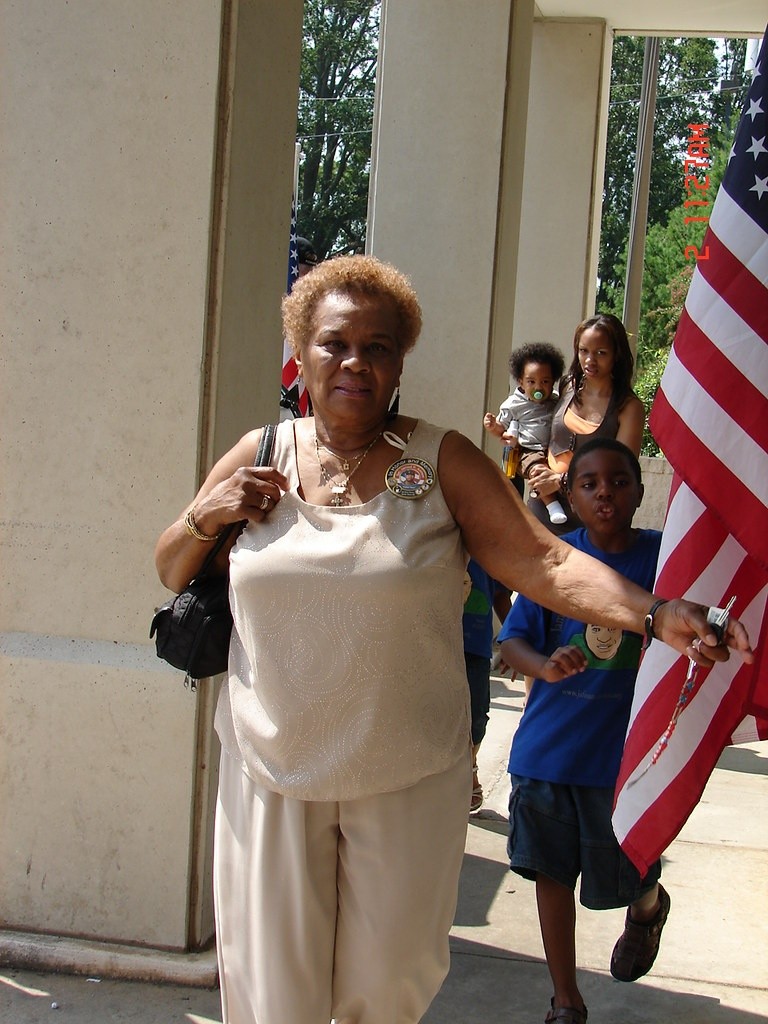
[644,599,668,650]
[185,504,225,540]
[560,472,567,491]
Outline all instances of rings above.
[530,489,540,498]
[260,497,268,509]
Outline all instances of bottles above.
[499,420,520,480]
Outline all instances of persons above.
[463,563,521,810]
[498,312,645,690]
[497,437,671,1024]
[153,254,754,1024]
[484,343,567,524]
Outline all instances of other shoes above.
[469,757,483,810]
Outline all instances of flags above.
[609,20,768,875]
[280,194,314,418]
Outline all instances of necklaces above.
[314,432,383,507]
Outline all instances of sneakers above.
[610,882,670,982]
[545,996,588,1024]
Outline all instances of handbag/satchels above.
[149,424,276,680]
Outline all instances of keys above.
[710,595,737,642]
[687,646,698,680]
